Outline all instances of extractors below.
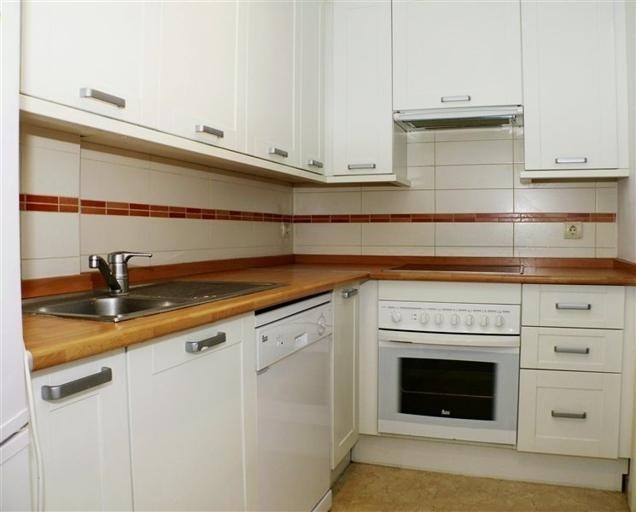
[403,116,519,135]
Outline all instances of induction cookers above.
[393,263,523,275]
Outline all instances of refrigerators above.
[253,299,333,510]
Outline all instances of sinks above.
[127,278,261,300]
[34,294,189,320]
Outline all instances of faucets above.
[89,248,152,295]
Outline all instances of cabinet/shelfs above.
[234,7,294,184]
[325,2,409,188]
[332,287,360,482]
[392,0,522,125]
[520,0,634,184]
[19,2,141,146]
[295,4,323,185]
[517,284,634,494]
[29,312,257,512]
[143,2,232,170]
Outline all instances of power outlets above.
[563,221,583,239]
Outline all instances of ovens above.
[377,300,520,442]
